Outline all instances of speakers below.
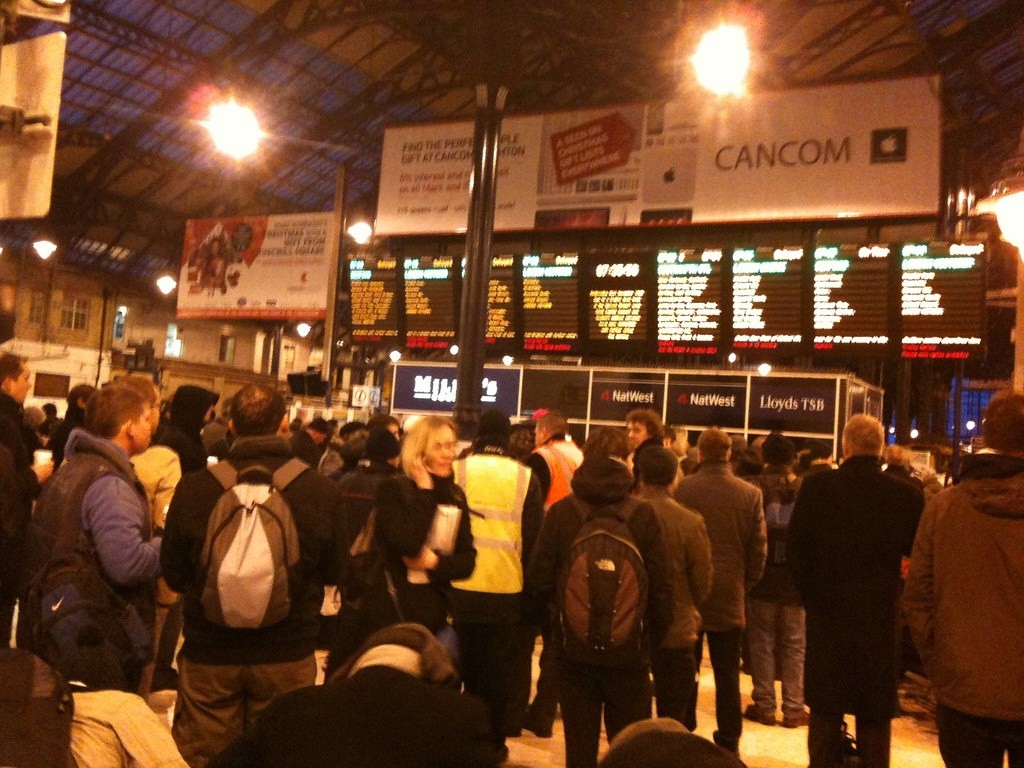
[287,370,324,398]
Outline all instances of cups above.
[206,456,219,468]
[162,504,170,529]
[33,450,52,468]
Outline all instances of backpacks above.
[200,458,310,629]
[323,507,403,654]
[558,493,652,654]
[28,468,152,693]
[1,643,73,766]
[747,475,804,566]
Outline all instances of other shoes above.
[783,709,810,727]
[713,732,738,751]
[523,704,559,738]
[745,705,777,725]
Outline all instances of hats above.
[365,428,400,462]
[984,391,1024,450]
[762,429,797,467]
[638,448,678,487]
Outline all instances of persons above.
[0,354,1024,767]
